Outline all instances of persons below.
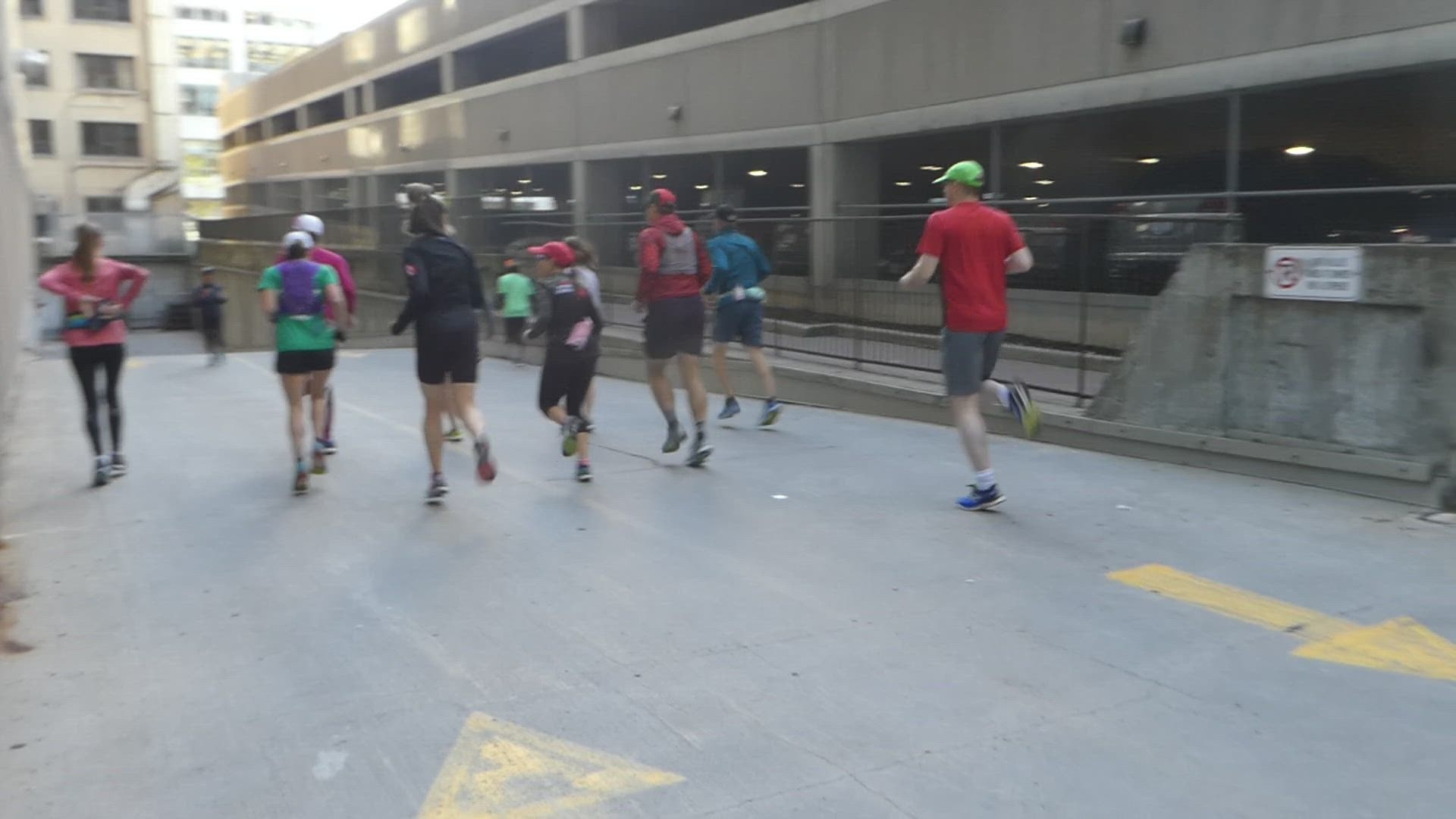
[631,189,715,466]
[256,230,347,496]
[274,215,357,453]
[701,204,782,427]
[901,161,1037,511]
[193,266,228,365]
[36,223,150,484]
[388,184,606,505]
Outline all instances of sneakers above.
[718,400,741,419]
[954,484,1006,510]
[663,425,688,454]
[574,466,594,480]
[1007,380,1041,437]
[560,415,583,457]
[757,399,784,426]
[684,437,714,468]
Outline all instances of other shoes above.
[111,449,126,476]
[442,426,463,441]
[311,453,327,476]
[291,472,309,494]
[473,436,496,479]
[423,477,448,506]
[314,438,337,453]
[93,456,108,484]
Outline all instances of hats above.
[291,214,326,237]
[527,242,575,269]
[643,189,677,209]
[714,205,737,223]
[408,194,458,237]
[931,160,985,189]
[280,231,314,249]
